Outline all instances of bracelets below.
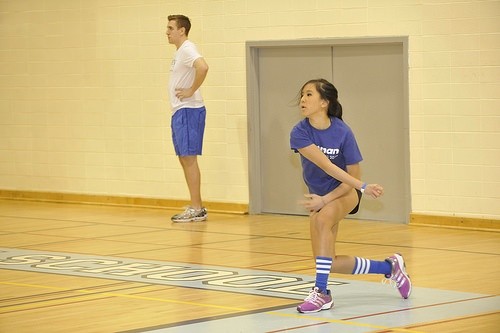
[321,196,326,205]
[361,182,367,193]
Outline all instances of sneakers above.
[297,289,333,313]
[203,208,208,221]
[386,253,412,298]
[172,210,204,222]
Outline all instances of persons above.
[166,15,209,223]
[288,78,412,313]
